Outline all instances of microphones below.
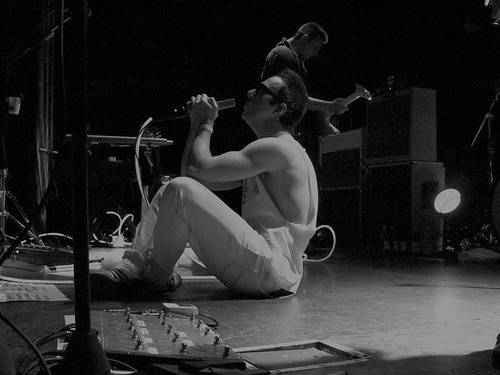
[166,99,236,120]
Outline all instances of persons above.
[89,68,318,301]
[260,22,349,248]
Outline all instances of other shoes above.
[121,271,182,297]
[88,265,142,293]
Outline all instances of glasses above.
[255,83,283,105]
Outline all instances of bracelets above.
[199,124,214,134]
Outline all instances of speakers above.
[364,89,437,162]
[316,126,365,187]
[315,187,366,249]
[367,164,447,254]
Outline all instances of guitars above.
[319,84,373,137]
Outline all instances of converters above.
[111,235,126,247]
